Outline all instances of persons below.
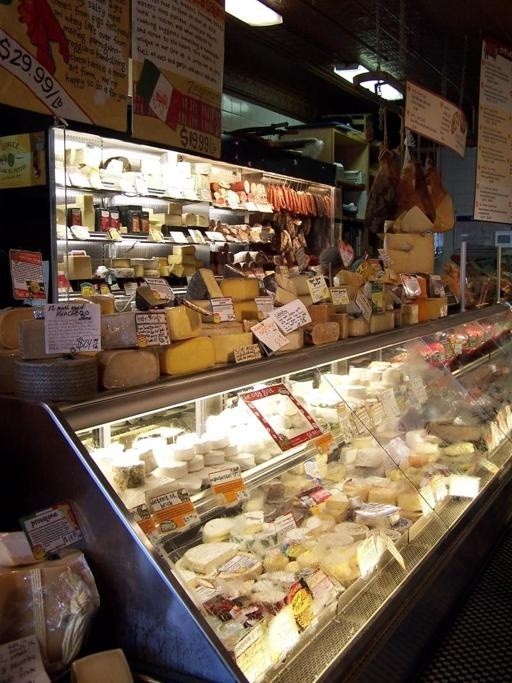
[319,240,363,276]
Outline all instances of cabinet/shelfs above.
[2,115,341,304]
[283,125,380,269]
[3,303,511,682]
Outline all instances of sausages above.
[266,185,332,217]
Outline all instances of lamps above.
[333,62,370,84]
[353,0,403,101]
[225,0,283,28]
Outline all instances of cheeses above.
[0,245,475,683]
